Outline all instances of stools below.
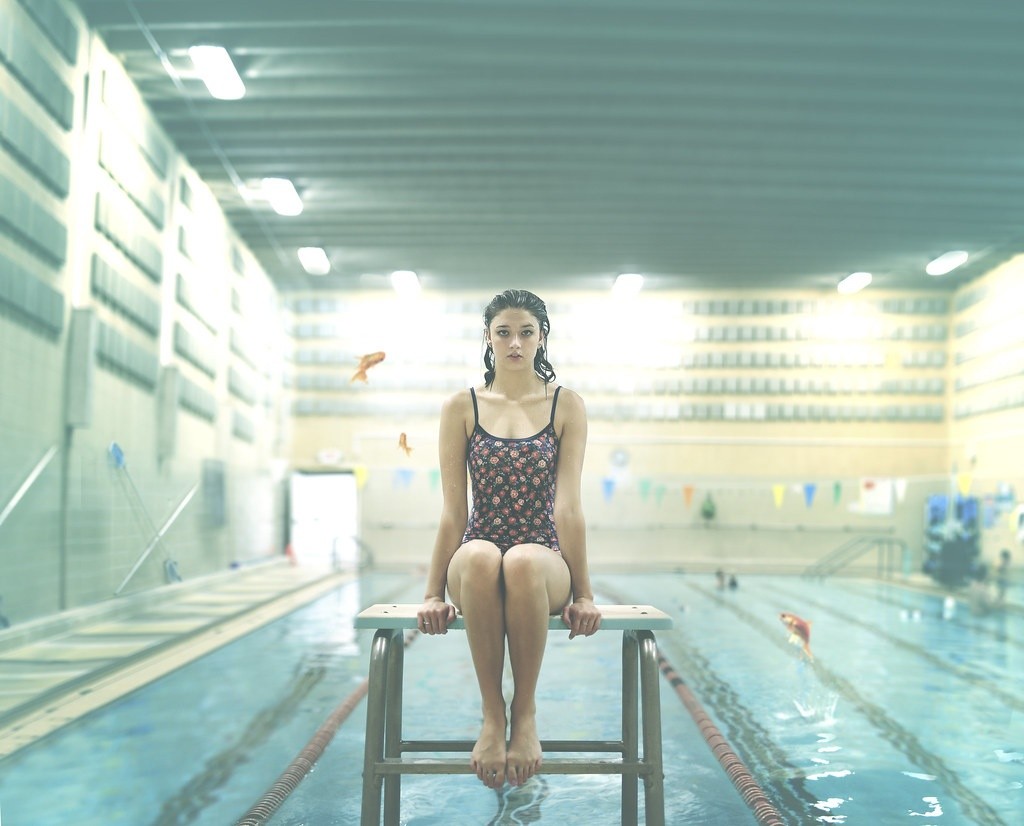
[352,601,677,826]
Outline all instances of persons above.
[415,288,602,789]
[963,549,1010,607]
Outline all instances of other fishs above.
[348,351,387,384]
[781,611,816,660]
[397,430,413,457]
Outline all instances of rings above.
[423,622,431,624]
[580,623,588,626]
